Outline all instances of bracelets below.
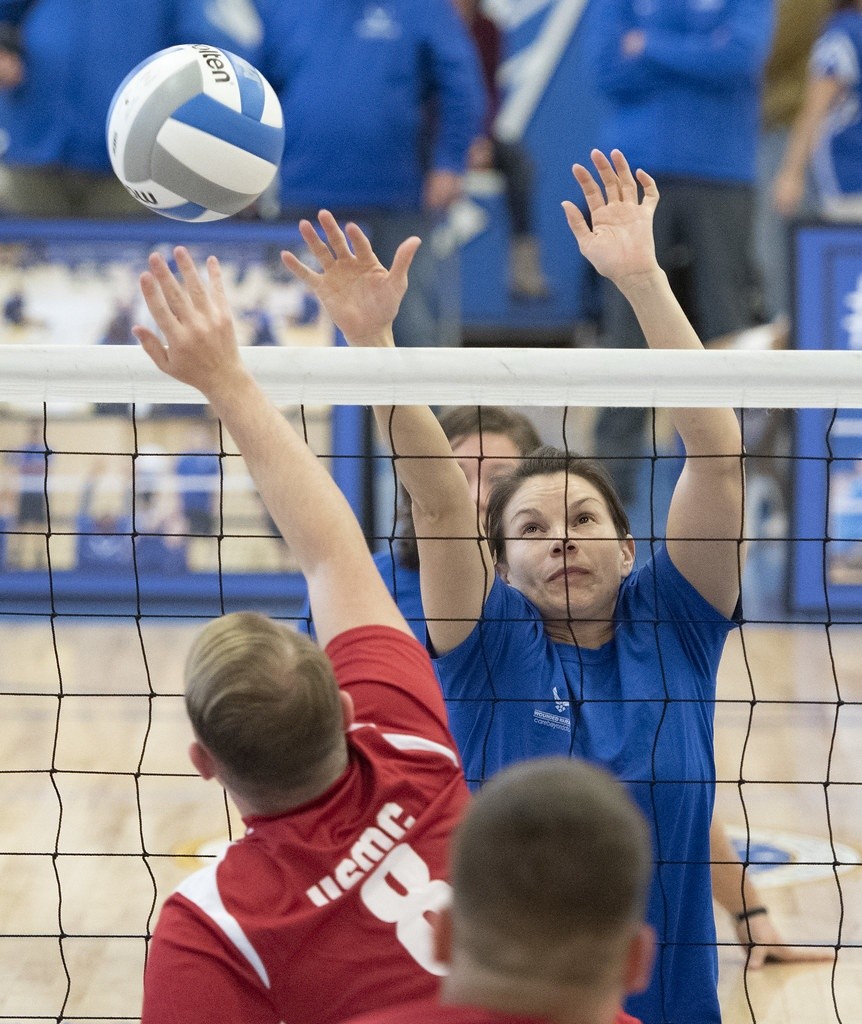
[736,908,766,922]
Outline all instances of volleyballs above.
[104,38,289,225]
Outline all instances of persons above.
[0,0,862,350]
[340,756,655,1024]
[280,146,837,1024]
[131,246,475,1024]
